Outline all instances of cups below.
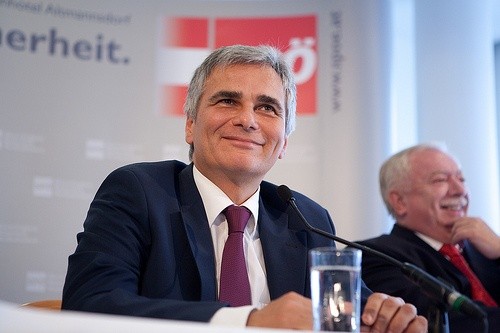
[308,247,362,333]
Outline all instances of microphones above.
[278,185,491,323]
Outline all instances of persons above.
[342,145,500,333]
[60,41,430,333]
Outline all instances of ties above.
[219,205,253,307]
[440,242,496,307]
